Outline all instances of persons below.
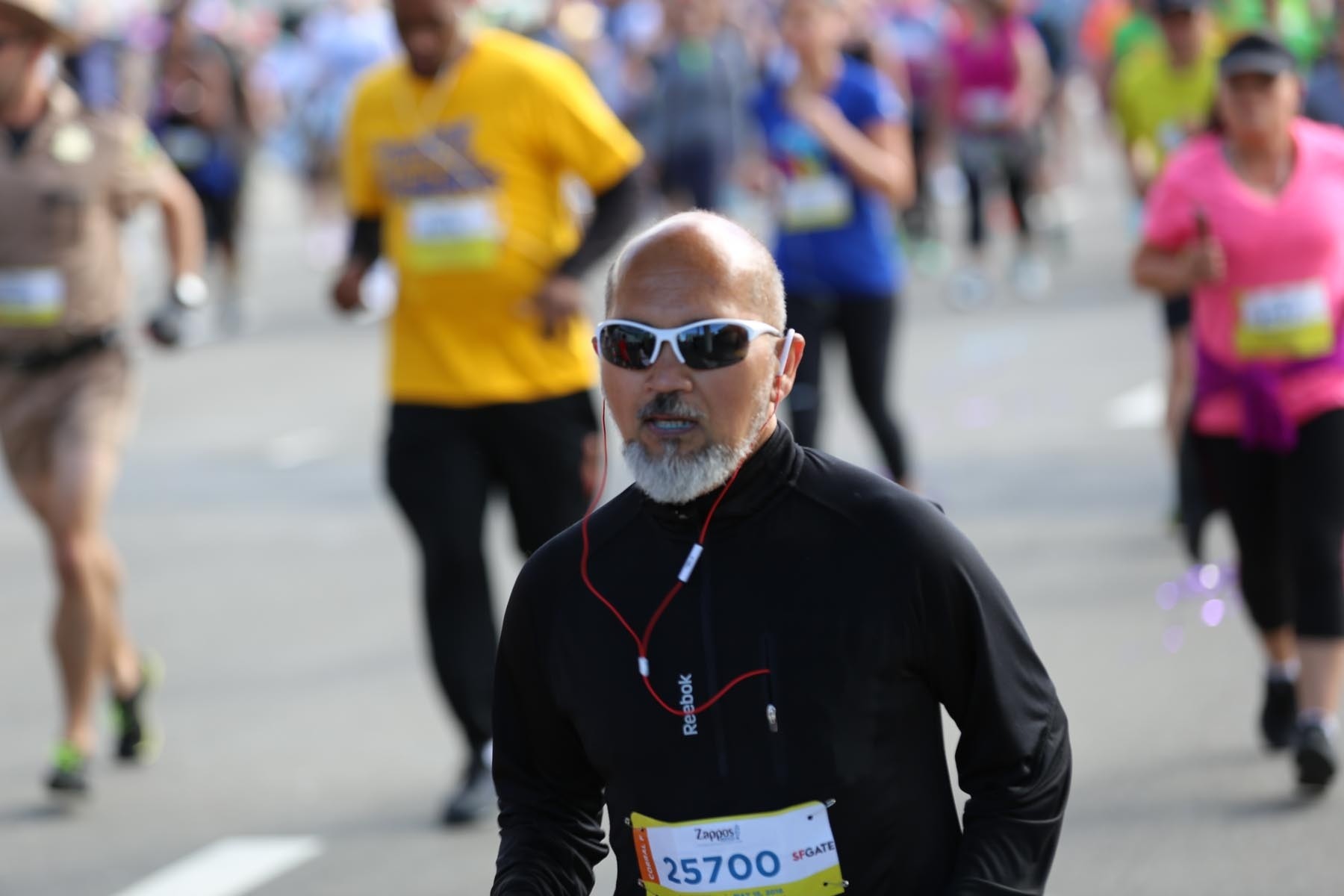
[333,0,646,827]
[1131,32,1343,794]
[60,0,1344,334]
[0,0,208,792]
[490,209,1074,896]
[736,1,915,488]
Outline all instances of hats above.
[1219,36,1292,78]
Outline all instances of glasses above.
[596,319,784,372]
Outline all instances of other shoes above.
[1261,674,1297,746]
[50,749,90,793]
[1296,727,1336,791]
[445,763,499,824]
[112,651,161,758]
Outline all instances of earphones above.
[776,327,796,376]
